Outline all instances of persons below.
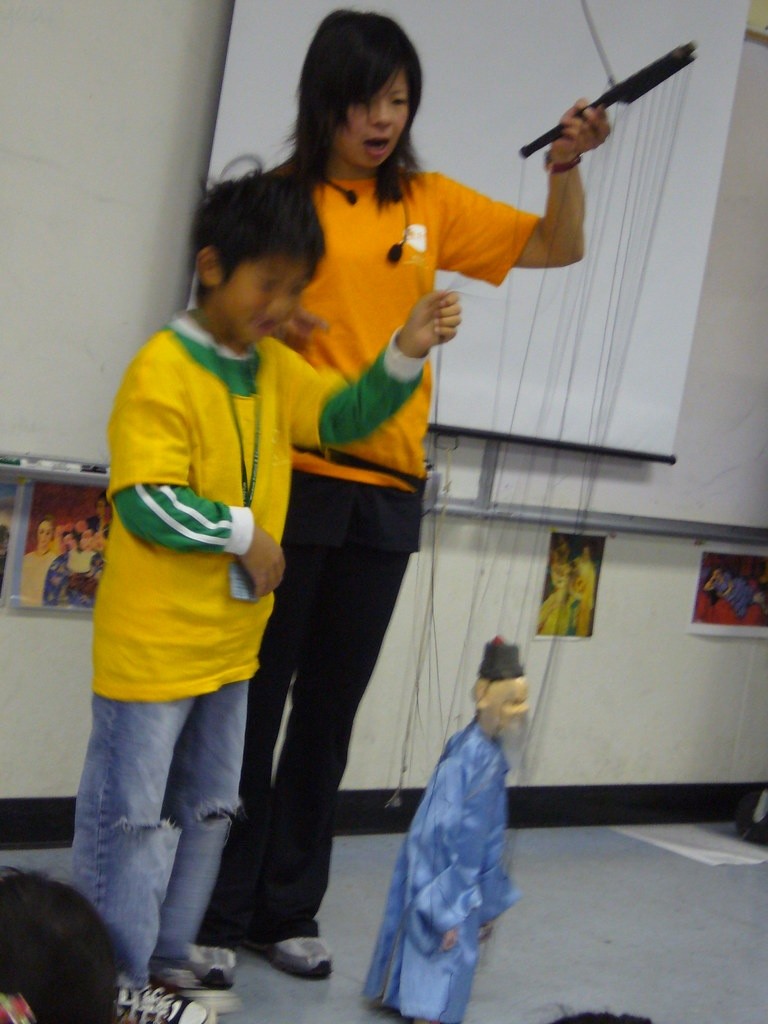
[67,155,464,1024]
[185,9,616,990]
[537,535,596,636]
[703,565,768,619]
[361,635,529,1024]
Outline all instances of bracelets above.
[543,150,581,174]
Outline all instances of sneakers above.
[155,947,233,990]
[117,983,217,1024]
[270,937,333,979]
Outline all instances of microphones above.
[384,196,408,262]
